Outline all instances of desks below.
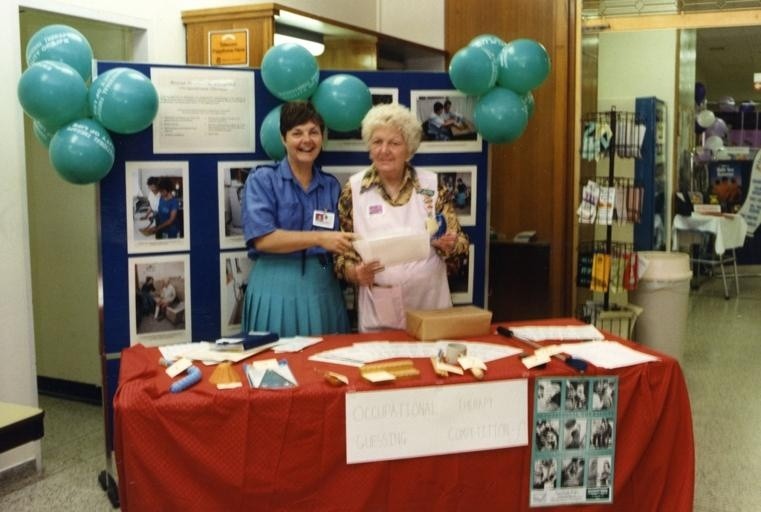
[103,319,698,511]
[671,208,751,301]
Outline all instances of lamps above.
[378,47,408,73]
[272,16,327,58]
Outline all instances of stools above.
[0,398,47,487]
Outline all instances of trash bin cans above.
[630,250,694,370]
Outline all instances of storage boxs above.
[401,306,495,343]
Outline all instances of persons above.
[428,99,465,141]
[533,380,613,490]
[239,99,361,336]
[334,103,470,333]
[137,177,182,240]
[141,276,176,320]
[440,175,468,209]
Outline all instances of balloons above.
[311,73,373,132]
[695,81,757,165]
[449,34,552,144]
[260,43,320,103]
[17,25,159,185]
[260,103,286,162]
